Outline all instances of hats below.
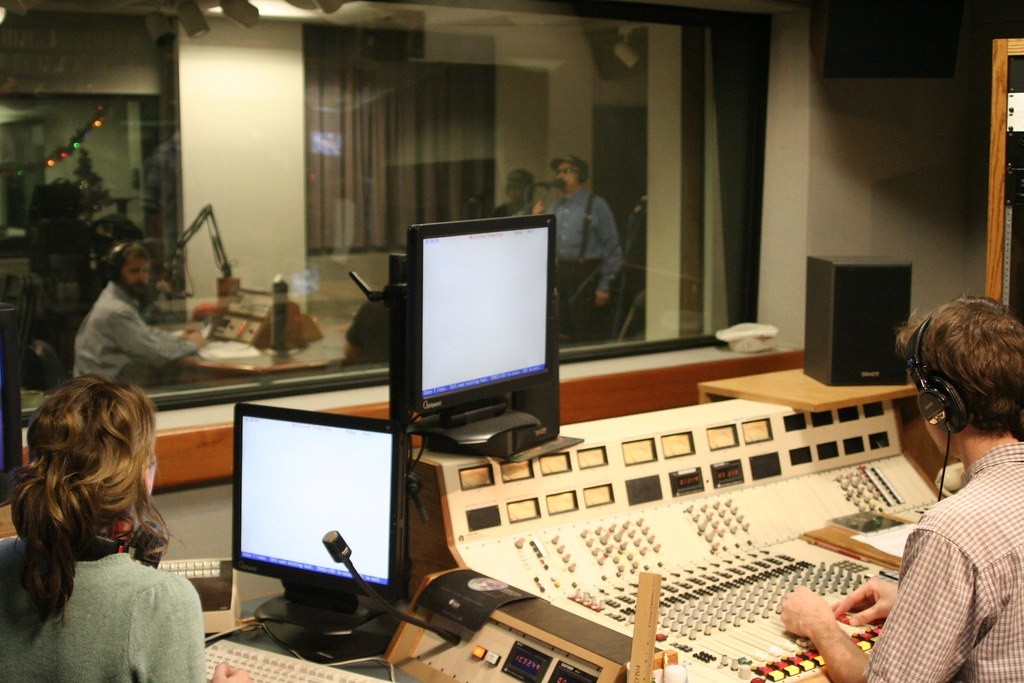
[550,154,589,183]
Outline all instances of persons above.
[71,244,205,379]
[0,380,251,683]
[492,157,621,338]
[778,296,1024,683]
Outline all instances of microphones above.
[321,530,459,645]
[155,280,188,300]
[534,178,565,188]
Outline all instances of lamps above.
[144,0,176,50]
[176,0,258,38]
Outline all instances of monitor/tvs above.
[230,403,407,634]
[412,213,557,461]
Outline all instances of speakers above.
[505,263,561,458]
[804,255,914,386]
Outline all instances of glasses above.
[556,167,578,173]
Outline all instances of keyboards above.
[203,639,393,683]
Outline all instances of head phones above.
[906,314,967,435]
[104,240,135,281]
[74,519,164,570]
